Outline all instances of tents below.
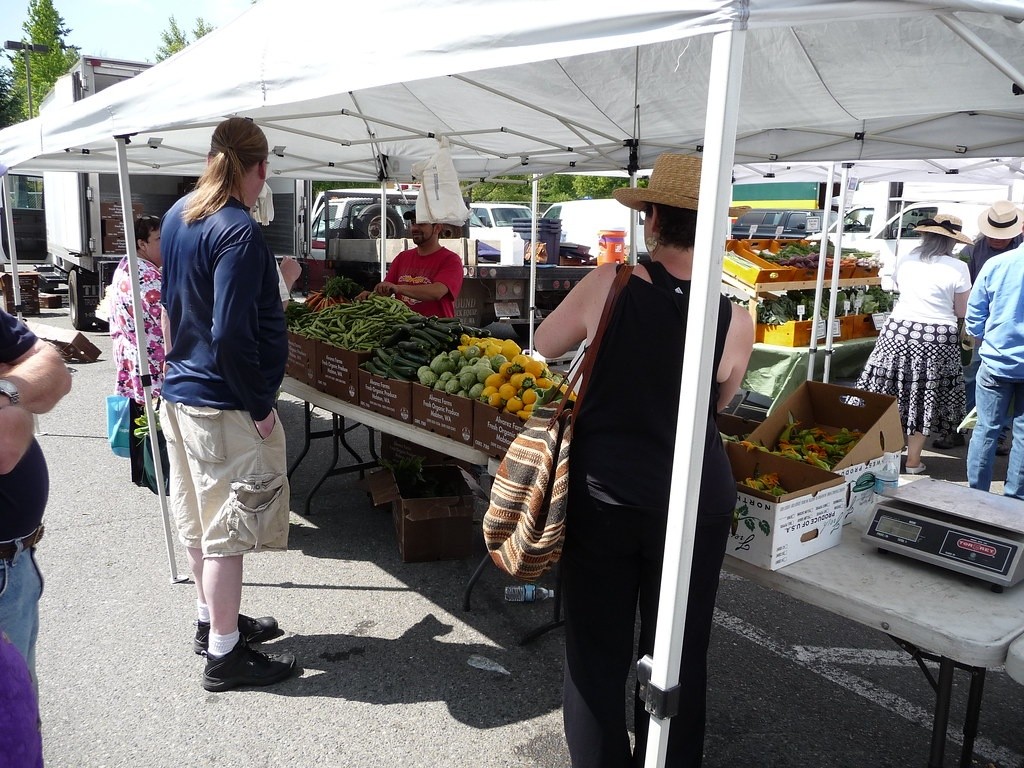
[0,0,1024,768]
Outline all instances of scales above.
[861,477,1024,593]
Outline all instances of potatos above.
[780,253,822,269]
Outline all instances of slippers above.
[906,462,927,473]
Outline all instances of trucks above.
[39,55,312,330]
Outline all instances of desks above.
[280,376,1024,768]
[734,338,877,415]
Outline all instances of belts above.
[0,523,44,558]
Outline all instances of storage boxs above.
[0,271,63,316]
[284,237,905,573]
[100,203,144,254]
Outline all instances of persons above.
[279,255,302,312]
[965,241,1024,500]
[0,307,72,700]
[109,215,165,483]
[160,118,289,691]
[534,153,755,768]
[355,209,463,318]
[961,200,1024,288]
[854,214,974,474]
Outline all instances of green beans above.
[350,292,408,315]
[285,301,416,352]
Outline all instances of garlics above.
[857,255,885,269]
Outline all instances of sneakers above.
[193,613,278,653]
[996,427,1013,454]
[933,432,965,449]
[201,632,296,692]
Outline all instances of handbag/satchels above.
[130,394,171,496]
[483,398,574,582]
[106,394,131,458]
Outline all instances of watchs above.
[0,380,19,406]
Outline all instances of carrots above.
[825,255,857,266]
[307,295,345,311]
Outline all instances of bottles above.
[873,452,898,504]
[504,584,554,602]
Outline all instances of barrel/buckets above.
[597,230,627,267]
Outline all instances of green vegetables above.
[756,285,895,326]
[133,406,162,438]
[758,241,874,261]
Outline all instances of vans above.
[313,188,652,261]
[732,196,997,259]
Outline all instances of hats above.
[912,214,975,246]
[403,208,437,225]
[612,153,751,217]
[978,201,1024,239]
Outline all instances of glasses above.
[136,214,159,233]
